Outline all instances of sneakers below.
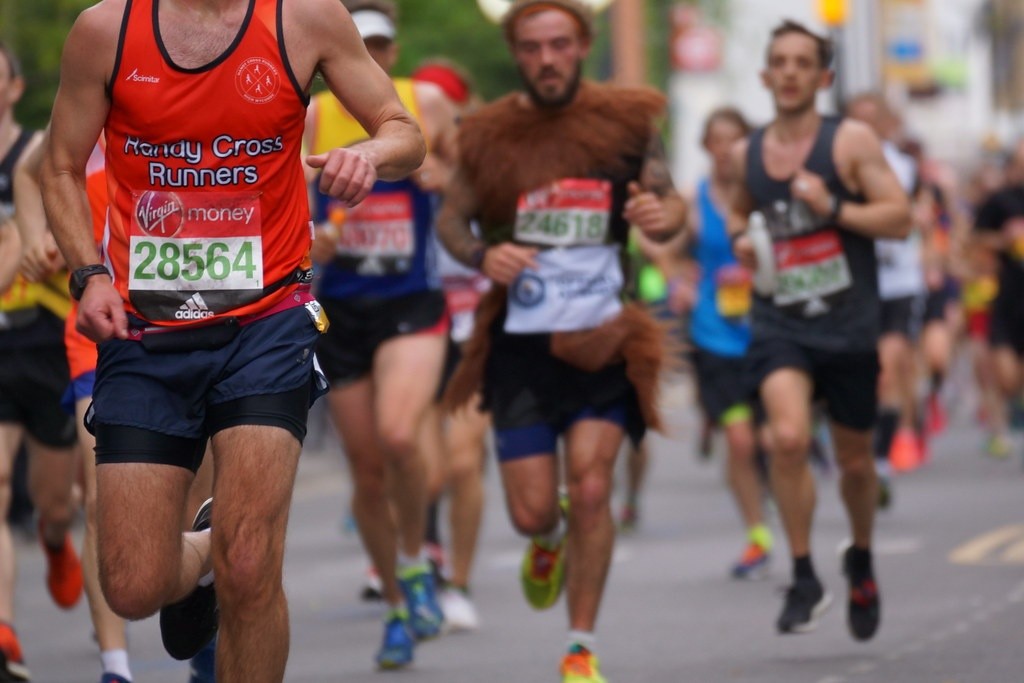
[160,500,219,660]
[778,577,824,634]
[734,525,775,579]
[374,610,413,668]
[520,495,571,611]
[837,537,882,643]
[396,560,444,641]
[0,621,29,683]
[33,508,84,610]
[559,643,606,683]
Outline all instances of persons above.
[0,38,222,683]
[299,0,1023,682]
[39,0,429,683]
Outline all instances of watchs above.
[67,263,114,298]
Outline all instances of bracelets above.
[828,196,842,222]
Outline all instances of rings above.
[793,178,807,190]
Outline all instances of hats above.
[348,9,398,44]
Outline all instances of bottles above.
[744,211,777,296]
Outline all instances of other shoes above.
[441,586,478,634]
[873,410,901,460]
[920,386,948,435]
[421,540,454,581]
[890,427,926,472]
[359,565,385,597]
